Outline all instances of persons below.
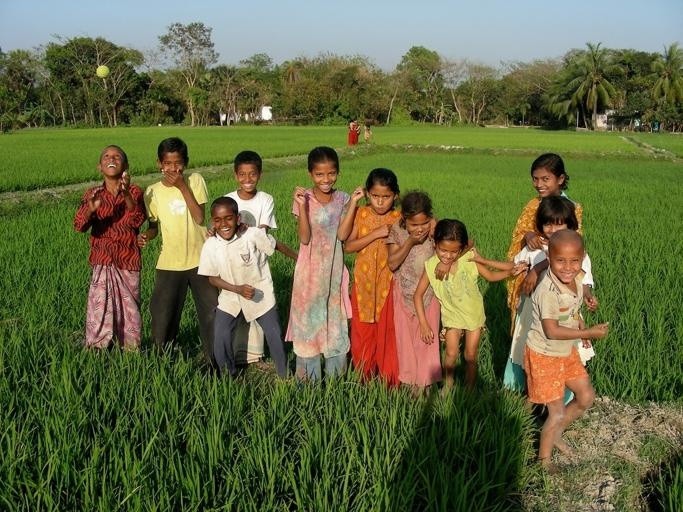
[136,138,218,376]
[194,198,300,382]
[633,119,640,132]
[388,190,474,401]
[530,231,609,466]
[414,220,523,395]
[344,166,405,391]
[347,116,361,146]
[72,144,147,356]
[282,147,365,390]
[363,120,374,145]
[220,150,277,367]
[467,193,599,409]
